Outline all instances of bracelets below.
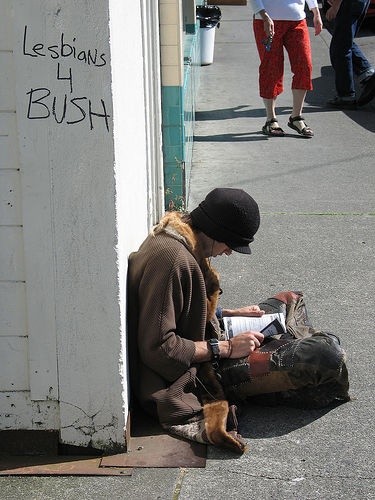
[227,339,233,358]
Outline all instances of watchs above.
[207,338,220,369]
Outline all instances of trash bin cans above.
[196,5,223,65]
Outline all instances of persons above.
[325,0,375,110]
[128,188,350,454]
[247,0,322,138]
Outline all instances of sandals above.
[262,118,286,137]
[288,116,314,136]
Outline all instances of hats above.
[190,188,260,255]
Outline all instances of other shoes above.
[326,96,356,110]
[357,71,375,107]
